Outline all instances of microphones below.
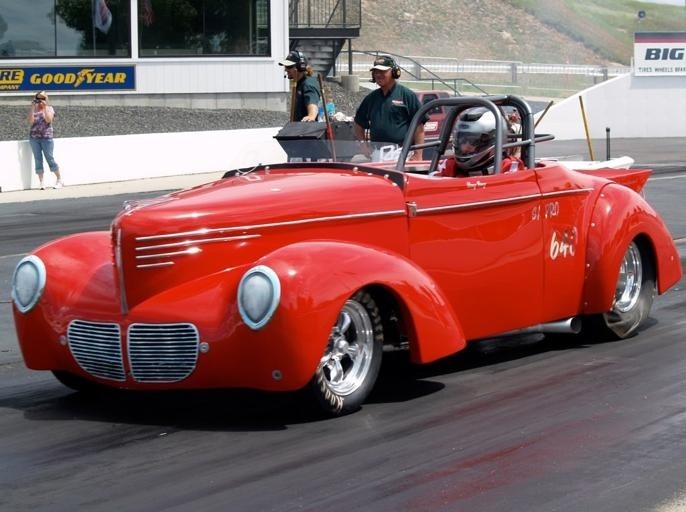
[283,70,287,78]
[369,79,376,83]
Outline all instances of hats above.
[278,52,308,66]
[370,56,397,71]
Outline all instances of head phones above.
[371,55,401,79]
[296,50,307,72]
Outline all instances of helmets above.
[452,106,509,172]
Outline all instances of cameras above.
[34,99,41,103]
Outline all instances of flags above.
[93,1,114,36]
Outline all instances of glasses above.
[285,66,293,69]
[458,137,481,146]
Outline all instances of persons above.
[325,92,336,118]
[27,89,63,189]
[277,50,322,123]
[353,55,429,161]
[427,103,526,180]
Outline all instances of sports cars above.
[11,92,685,414]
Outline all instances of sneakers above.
[39,180,63,190]
